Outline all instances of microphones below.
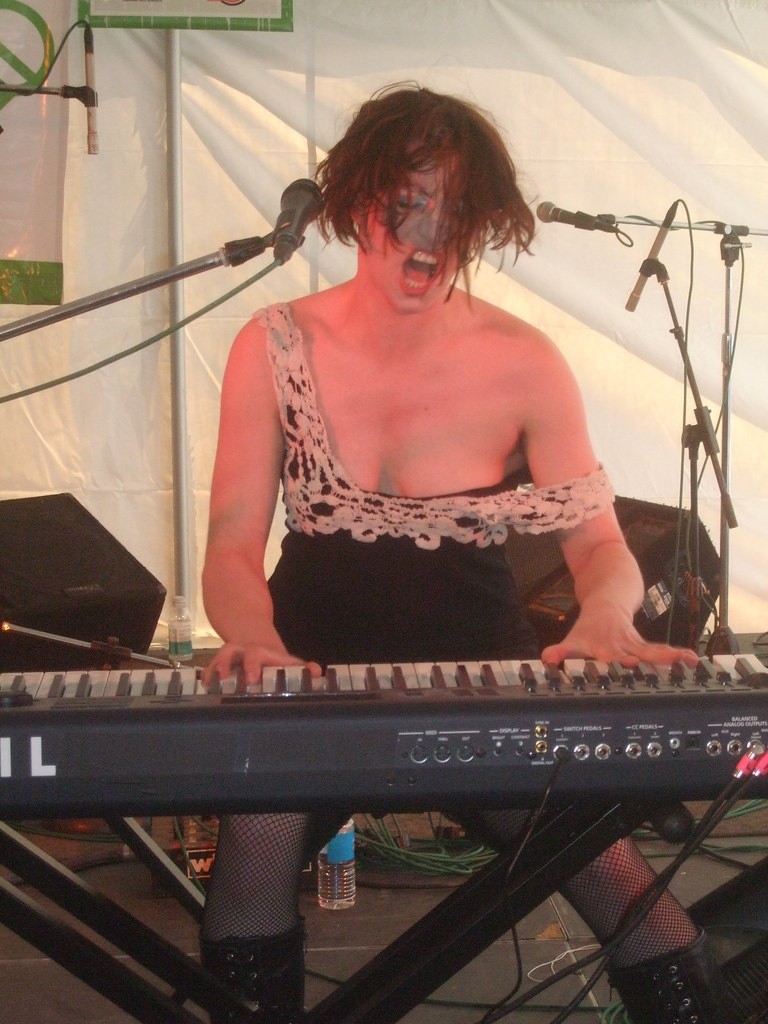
[626,201,678,312]
[274,179,323,265]
[537,202,619,233]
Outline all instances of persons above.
[198,88,720,1024]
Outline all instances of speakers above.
[685,855,768,1024]
[0,492,168,672]
[502,494,724,651]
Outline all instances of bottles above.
[169,596,193,661]
[318,819,356,911]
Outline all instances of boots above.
[199,916,306,1024]
[608,922,737,1024]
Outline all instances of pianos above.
[0,651,768,821]
